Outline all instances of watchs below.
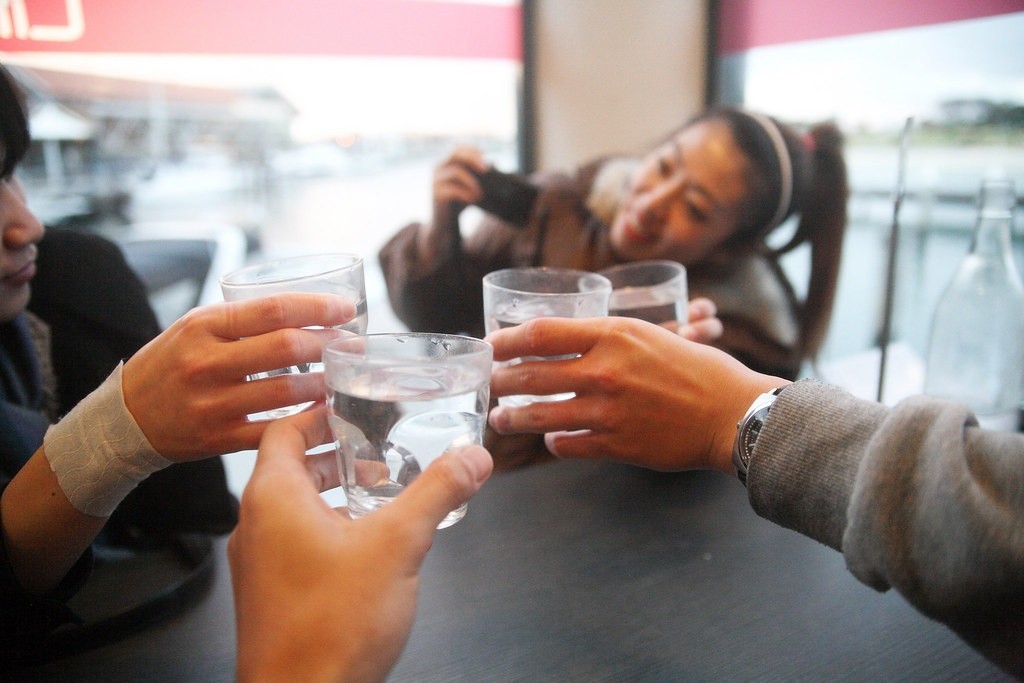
[727,384,794,490]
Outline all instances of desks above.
[0,450,1024,683]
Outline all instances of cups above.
[319,329,496,534]
[481,264,610,415]
[219,255,370,429]
[578,253,690,336]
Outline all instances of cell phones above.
[465,164,541,227]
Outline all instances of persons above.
[471,313,1024,680]
[379,106,849,478]
[0,57,364,617]
